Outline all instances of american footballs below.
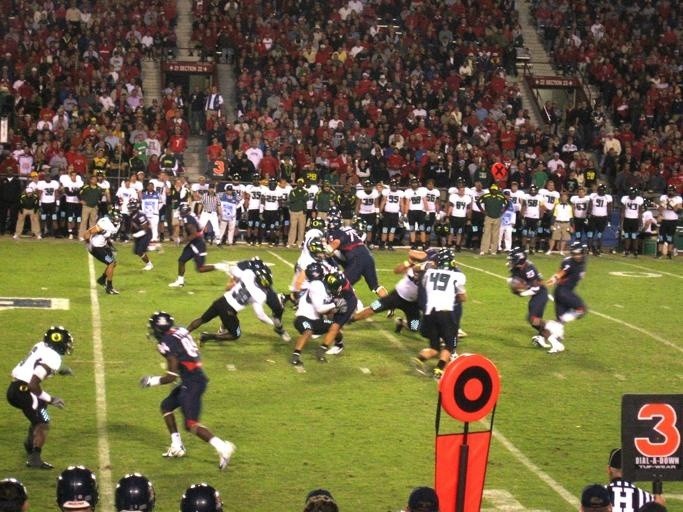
[511,282,526,291]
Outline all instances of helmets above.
[180,482,224,512]
[56,465,99,511]
[249,256,273,288]
[2,166,677,200]
[305,207,344,295]
[44,327,74,357]
[503,247,527,271]
[146,312,175,344]
[570,239,587,264]
[109,202,140,223]
[178,204,191,218]
[113,472,156,512]
[427,249,456,274]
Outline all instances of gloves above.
[59,367,72,376]
[335,298,348,314]
[39,390,65,409]
[160,360,169,372]
[139,375,162,388]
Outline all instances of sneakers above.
[528,314,578,354]
[141,261,154,271]
[199,330,208,349]
[96,278,119,296]
[167,281,186,288]
[364,241,680,260]
[154,243,164,255]
[27,458,55,470]
[386,299,470,378]
[223,261,235,279]
[22,439,35,454]
[274,317,345,369]
[1,230,303,249]
[218,441,236,470]
[161,445,187,460]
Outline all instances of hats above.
[406,484,441,510]
[580,482,611,508]
[304,486,337,506]
[634,500,668,512]
[608,447,624,469]
[0,476,27,500]
